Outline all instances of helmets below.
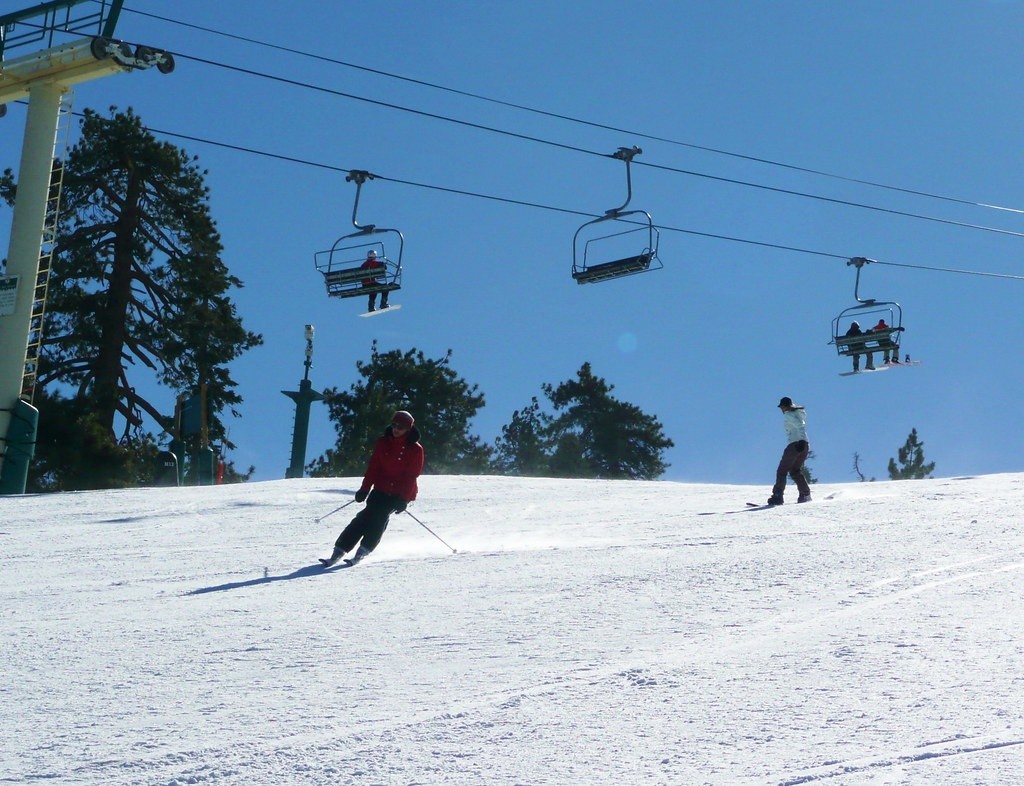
[368,251,378,258]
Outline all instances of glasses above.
[392,422,406,430]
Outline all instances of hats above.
[778,397,792,408]
[851,321,859,328]
[393,411,414,428]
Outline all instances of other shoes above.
[885,361,890,364]
[768,496,784,504]
[798,494,811,502]
[865,366,876,371]
[891,358,901,364]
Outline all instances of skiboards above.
[318,555,361,568]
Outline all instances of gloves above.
[394,500,406,514]
[356,490,368,502]
[795,440,806,451]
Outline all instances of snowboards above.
[359,304,402,318]
[838,365,890,377]
[745,498,814,507]
[879,360,921,369]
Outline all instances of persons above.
[360,250,390,312]
[845,321,875,372]
[872,318,901,365]
[318,411,424,567]
[767,397,811,504]
